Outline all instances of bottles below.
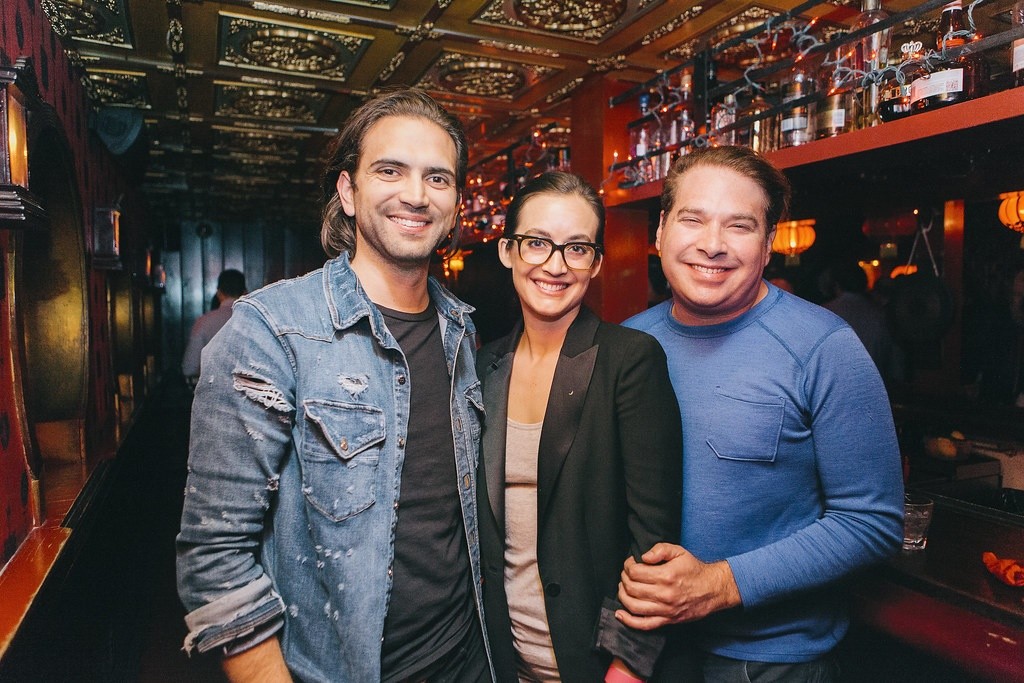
[464,150,572,237]
[780,0,1024,150]
[623,59,780,185]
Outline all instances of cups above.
[902,492,934,551]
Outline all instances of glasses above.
[509,233,602,269]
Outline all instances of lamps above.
[131,245,156,286]
[0,54,50,235]
[151,264,167,288]
[92,191,130,273]
[996,185,1024,243]
[772,216,819,257]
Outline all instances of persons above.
[175,89,907,683]
